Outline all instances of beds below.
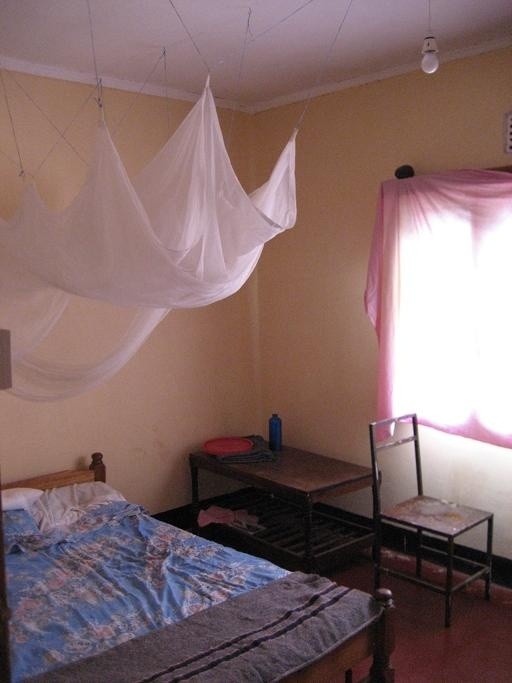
[0,453,397,682]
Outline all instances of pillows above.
[1,479,144,553]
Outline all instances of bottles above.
[268,412,282,451]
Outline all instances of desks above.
[186,441,381,578]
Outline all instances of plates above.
[203,435,253,454]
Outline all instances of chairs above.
[366,411,495,628]
